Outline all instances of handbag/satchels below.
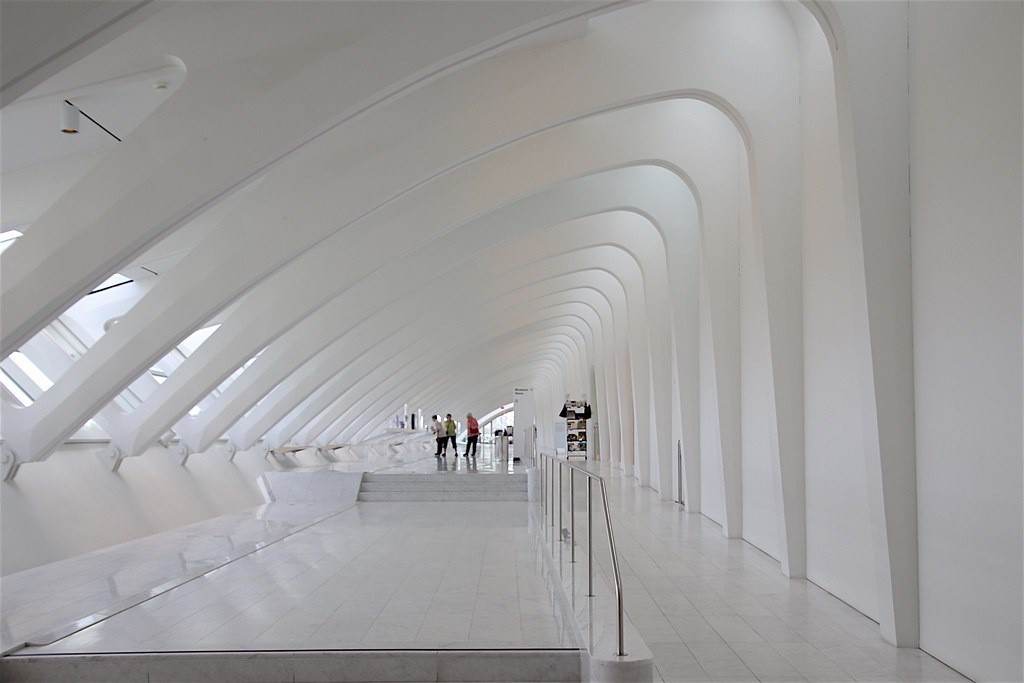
[470,428,479,434]
[445,420,451,435]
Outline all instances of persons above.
[462,413,481,457]
[443,414,458,457]
[430,414,447,456]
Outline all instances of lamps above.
[58,100,80,135]
